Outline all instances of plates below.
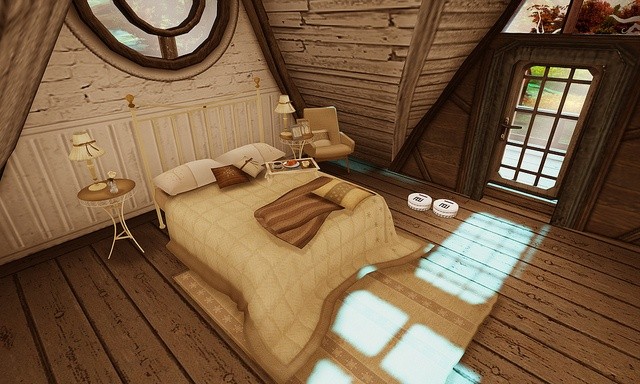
[283,162,300,168]
[271,166,284,170]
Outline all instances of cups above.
[273,161,282,169]
